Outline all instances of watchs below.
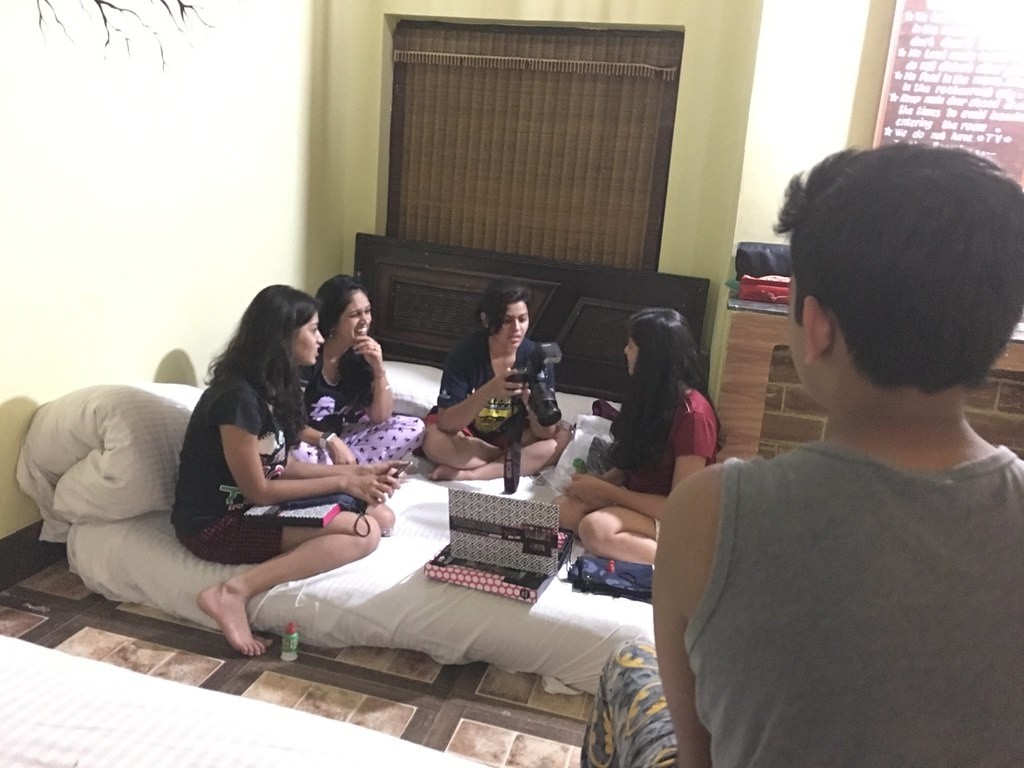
[320,431,337,448]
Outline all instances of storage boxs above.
[425,489,574,605]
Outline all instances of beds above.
[69,232,711,695]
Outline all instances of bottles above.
[279,622,299,661]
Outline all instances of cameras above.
[504,341,563,427]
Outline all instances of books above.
[243,501,343,528]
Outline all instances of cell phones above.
[386,462,413,486]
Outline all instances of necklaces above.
[321,368,341,387]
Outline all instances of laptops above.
[574,556,653,601]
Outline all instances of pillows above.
[375,360,623,425]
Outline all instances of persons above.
[556,306,718,568]
[651,141,1024,768]
[169,285,413,655]
[421,284,573,481]
[292,274,424,487]
